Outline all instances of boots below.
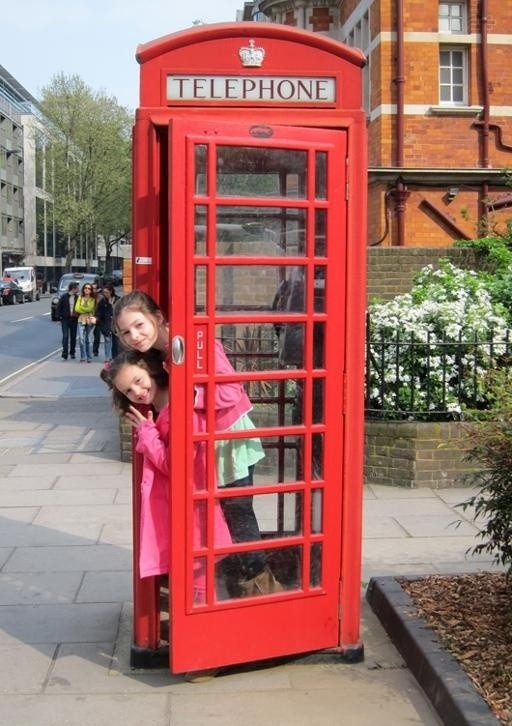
[237,565,286,597]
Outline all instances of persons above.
[90,283,102,358]
[111,289,283,600]
[74,284,96,362]
[100,350,232,684]
[96,284,123,364]
[57,281,80,359]
[271,267,324,484]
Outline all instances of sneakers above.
[62,353,98,363]
[185,667,220,682]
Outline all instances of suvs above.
[50,272,103,322]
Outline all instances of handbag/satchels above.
[72,311,80,319]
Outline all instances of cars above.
[0,280,26,306]
[100,269,123,286]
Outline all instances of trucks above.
[3,266,43,302]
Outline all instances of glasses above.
[84,287,91,290]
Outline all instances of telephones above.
[269,258,326,365]
